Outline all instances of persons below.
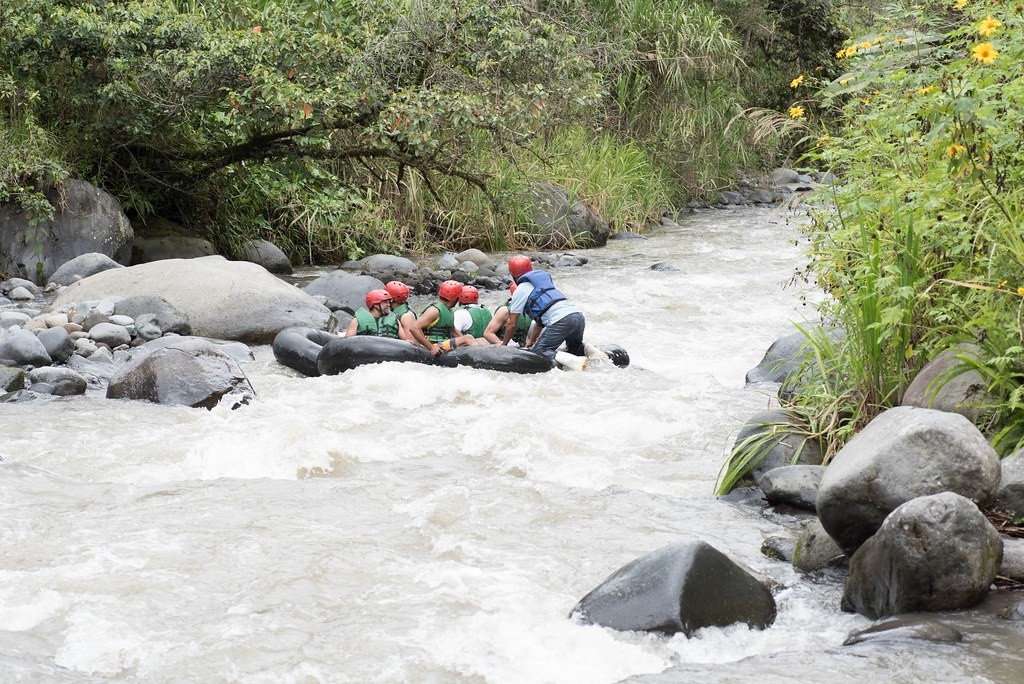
[345,290,408,340]
[484,282,531,345]
[384,281,421,346]
[409,280,491,357]
[501,255,608,372]
[453,285,492,338]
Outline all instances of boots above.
[584,344,609,365]
[554,350,589,373]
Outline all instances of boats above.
[273,326,630,378]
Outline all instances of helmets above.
[366,289,392,309]
[439,280,463,302]
[384,281,409,304]
[510,283,517,293]
[459,286,478,304]
[508,255,533,277]
